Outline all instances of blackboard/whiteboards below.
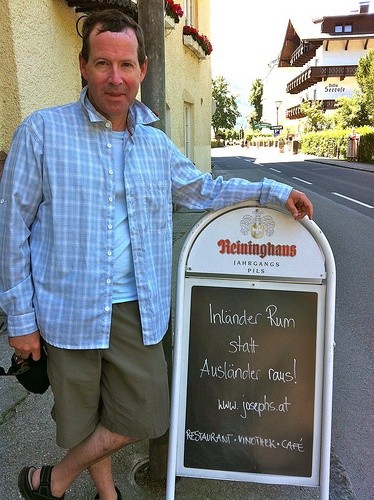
[175,277,325,490]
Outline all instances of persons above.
[0,10,313,500]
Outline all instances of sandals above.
[95,486,122,500]
[18,465,65,500]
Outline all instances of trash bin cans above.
[345,134,360,163]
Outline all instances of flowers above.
[183,25,213,55]
[165,0,184,23]
